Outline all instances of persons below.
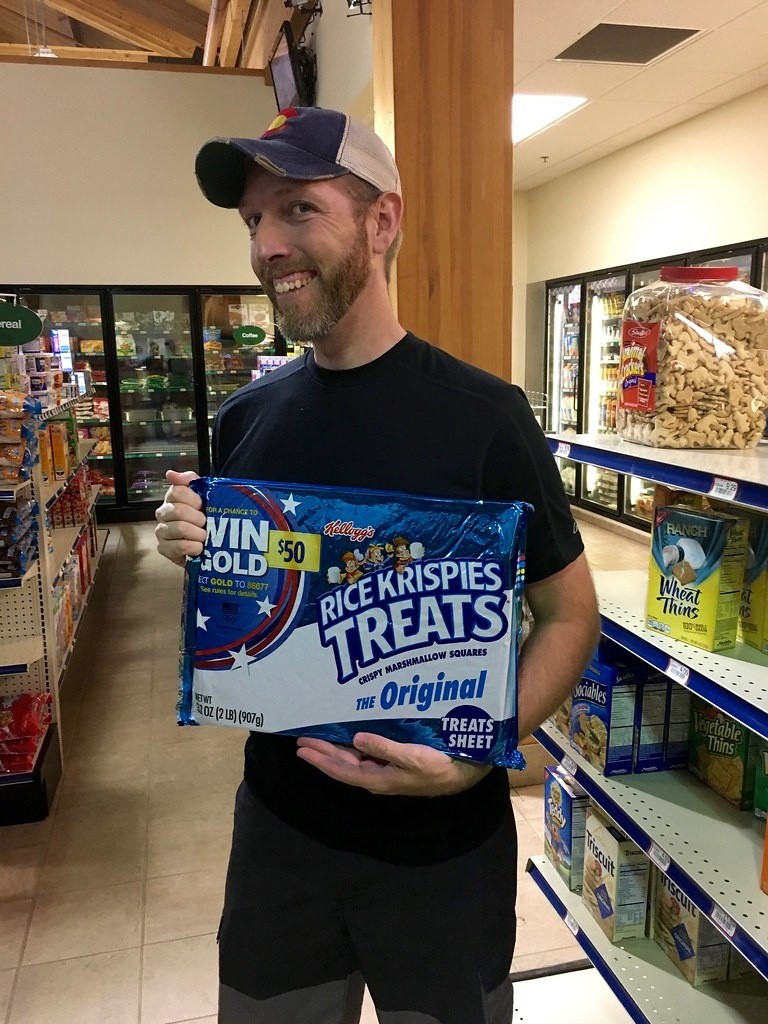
[143,342,166,436]
[165,339,195,442]
[154,105,600,1024]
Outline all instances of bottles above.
[597,290,626,434]
[561,302,579,423]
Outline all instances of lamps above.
[33,1,59,58]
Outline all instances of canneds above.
[21,338,80,410]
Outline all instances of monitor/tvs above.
[268,20,307,112]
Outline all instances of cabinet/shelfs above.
[510,239,768,1022]
[1,283,314,829]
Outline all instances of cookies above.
[548,696,608,775]
[694,745,744,802]
[615,289,768,450]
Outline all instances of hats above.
[194,105,403,210]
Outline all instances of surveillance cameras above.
[284,0,309,7]
